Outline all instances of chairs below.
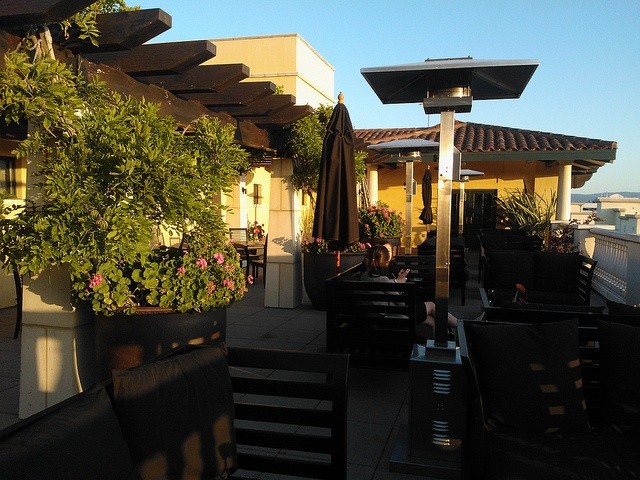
[229,228,259,279]
[250,234,268,288]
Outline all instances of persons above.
[360,245,458,341]
[378,240,398,279]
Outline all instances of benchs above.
[471,228,542,283]
[418,234,468,307]
[455,305,627,479]
[479,247,597,305]
[2,338,351,480]
[321,251,450,372]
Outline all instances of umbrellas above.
[418,165,434,233]
[311,91,360,274]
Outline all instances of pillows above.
[594,299,640,438]
[530,249,582,296]
[112,341,240,480]
[483,249,533,292]
[463,318,593,434]
[1,386,136,479]
